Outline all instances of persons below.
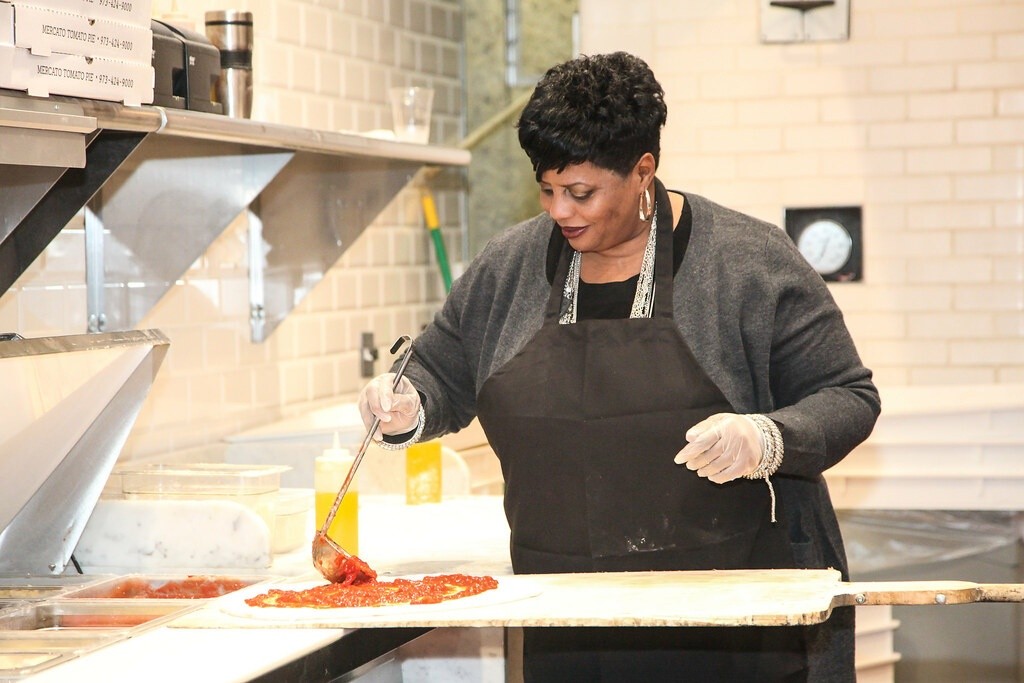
[360,51,882,682]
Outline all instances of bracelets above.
[743,413,784,480]
[373,404,426,451]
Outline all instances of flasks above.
[204,9,256,120]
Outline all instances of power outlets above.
[362,332,375,376]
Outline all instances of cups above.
[388,85,435,145]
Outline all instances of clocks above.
[786,205,864,284]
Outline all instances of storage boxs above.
[0,0,156,109]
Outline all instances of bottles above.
[315,432,360,560]
[405,438,442,505]
